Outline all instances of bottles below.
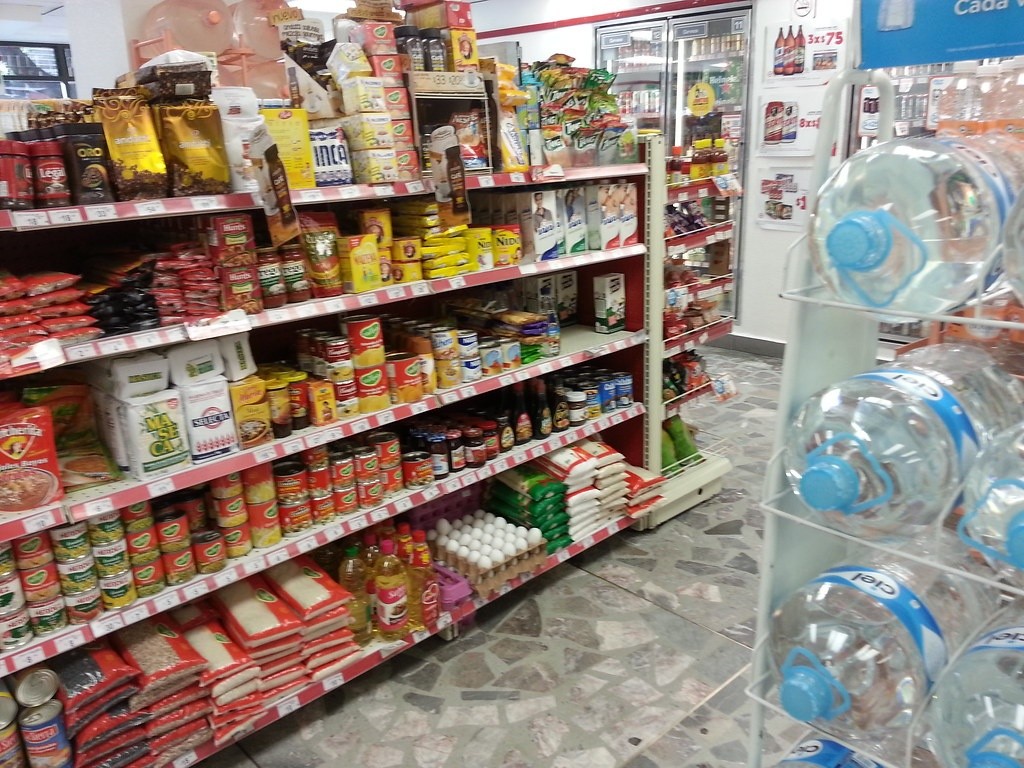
[962,422,1024,591]
[771,730,887,768]
[859,56,1024,140]
[690,138,711,179]
[692,33,745,56]
[510,378,554,445]
[712,139,730,176]
[926,594,1024,768]
[339,520,440,643]
[773,24,806,76]
[775,526,1002,742]
[670,146,683,188]
[392,23,447,73]
[444,146,468,215]
[782,341,1024,540]
[540,288,560,357]
[264,144,297,227]
[809,130,1024,324]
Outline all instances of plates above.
[1002,184,1024,305]
[434,196,452,203]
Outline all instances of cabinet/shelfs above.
[748,0,1024,768]
[593,10,751,318]
[0,134,737,768]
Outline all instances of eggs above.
[426,510,542,585]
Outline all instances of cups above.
[432,180,450,195]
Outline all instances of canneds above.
[260,304,634,540]
[0,459,282,768]
[280,244,310,301]
[420,28,447,72]
[394,25,425,71]
[27,141,71,207]
[256,247,287,308]
[692,33,743,55]
[782,102,798,142]
[613,89,660,114]
[0,140,35,209]
[764,101,784,144]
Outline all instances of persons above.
[367,217,414,282]
[555,190,586,227]
[533,192,553,232]
